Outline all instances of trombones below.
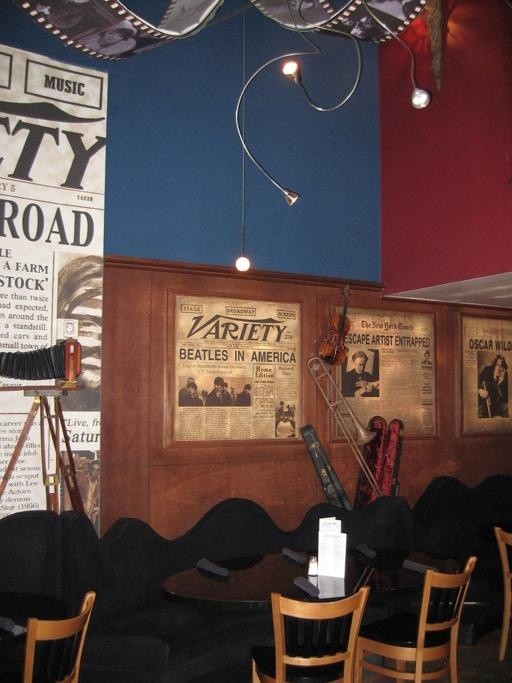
[307,356,383,497]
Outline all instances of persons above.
[343,351,379,397]
[79,460,100,524]
[478,354,508,418]
[179,377,251,406]
[276,401,295,437]
[421,351,432,364]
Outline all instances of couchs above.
[365,474,512,647]
[0,510,97,683]
[97,498,369,682]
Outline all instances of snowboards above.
[354,415,404,502]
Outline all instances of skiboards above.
[301,426,353,510]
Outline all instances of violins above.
[318,285,351,365]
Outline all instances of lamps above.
[233,0,430,272]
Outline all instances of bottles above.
[308,575,316,588]
[306,555,318,576]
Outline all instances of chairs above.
[353,556,478,683]
[23,590,96,683]
[250,585,371,683]
[494,527,512,663]
